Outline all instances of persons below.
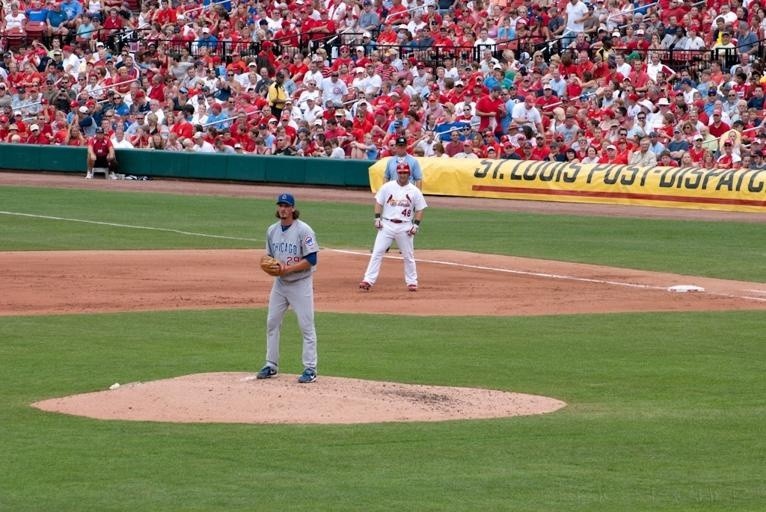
[86,127,118,178]
[381,138,424,254]
[256,193,320,383]
[1,1,765,170]
[358,164,428,292]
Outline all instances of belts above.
[385,217,413,224]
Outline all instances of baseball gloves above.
[261,256,283,276]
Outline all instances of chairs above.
[0,0,766,168]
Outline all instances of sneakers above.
[359,281,372,291]
[297,370,318,384]
[85,172,94,179]
[258,366,278,378]
[109,172,118,180]
[407,284,417,291]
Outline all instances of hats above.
[436,137,763,156]
[0,2,765,134]
[396,138,407,146]
[276,193,295,206]
[397,163,411,174]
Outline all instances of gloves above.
[408,225,418,236]
[374,218,383,230]
